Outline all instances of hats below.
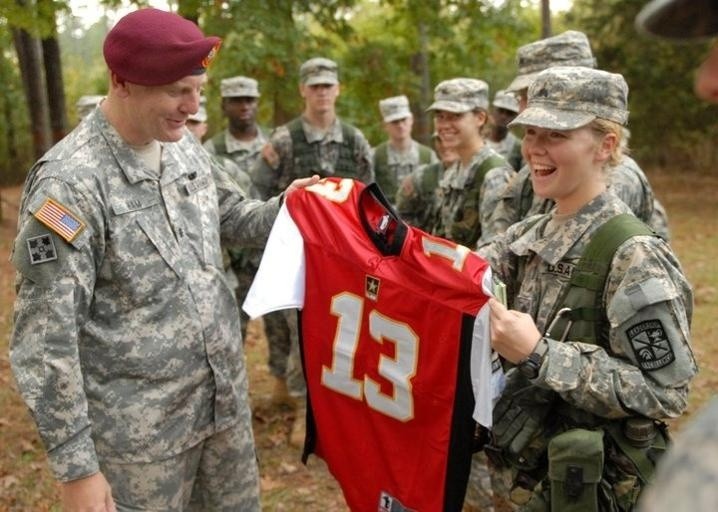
[74,94,105,122]
[505,29,595,93]
[634,0,717,40]
[101,7,223,88]
[220,75,261,100]
[188,89,207,124]
[379,93,414,123]
[506,65,631,141]
[299,57,339,88]
[422,77,490,116]
[493,90,521,116]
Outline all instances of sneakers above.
[272,376,298,409]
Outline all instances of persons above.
[9,7,320,511]
[185,60,372,448]
[77,95,103,124]
[462,28,697,511]
[374,77,516,512]
